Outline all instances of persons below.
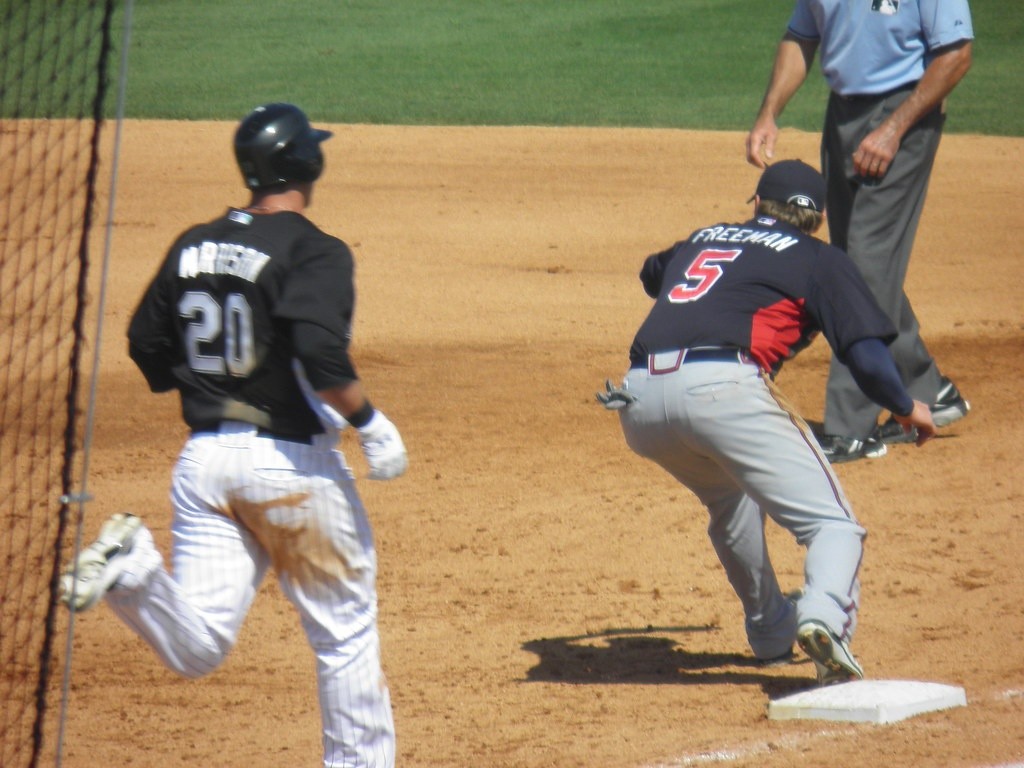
[745,0,976,461]
[52,103,409,768]
[618,158,935,683]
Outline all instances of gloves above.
[361,411,408,481]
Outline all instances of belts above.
[630,346,741,370]
[191,419,313,444]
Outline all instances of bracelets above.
[349,401,374,428]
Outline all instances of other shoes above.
[760,588,803,668]
[796,621,864,686]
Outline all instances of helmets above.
[235,103,331,189]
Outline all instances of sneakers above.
[878,376,971,444]
[59,515,161,610]
[818,434,887,464]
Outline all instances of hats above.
[748,160,827,210]
